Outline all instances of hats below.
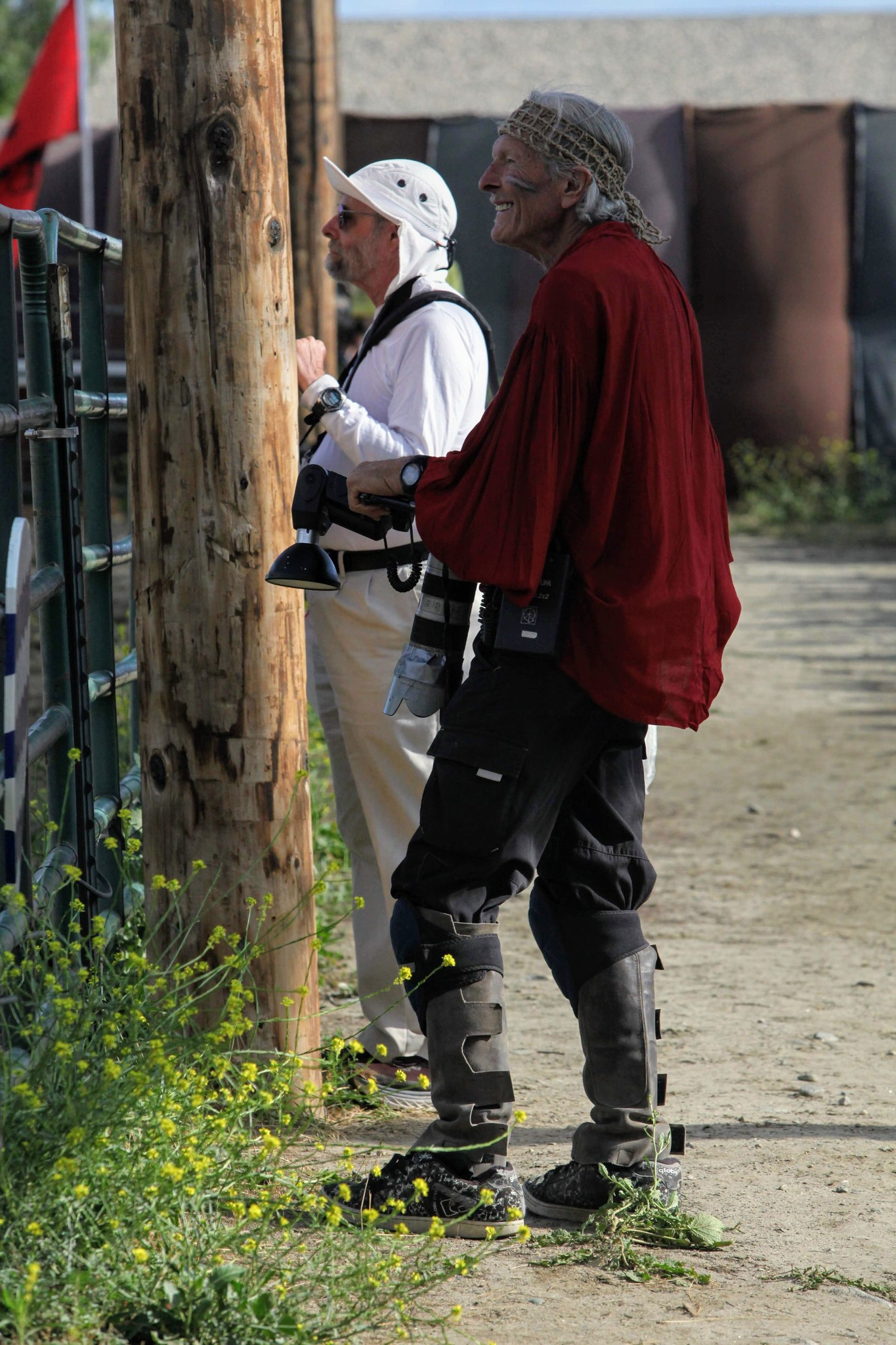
[322,155,458,248]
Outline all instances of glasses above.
[337,203,375,229]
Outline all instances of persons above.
[299,156,491,1111]
[322,91,742,1239]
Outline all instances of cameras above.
[384,550,478,718]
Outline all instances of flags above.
[0,0,88,264]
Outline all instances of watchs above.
[302,385,349,427]
[396,454,431,497]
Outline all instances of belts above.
[322,541,429,574]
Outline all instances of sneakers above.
[319,1043,378,1105]
[352,1056,435,1111]
[522,1158,682,1227]
[319,1153,525,1239]
